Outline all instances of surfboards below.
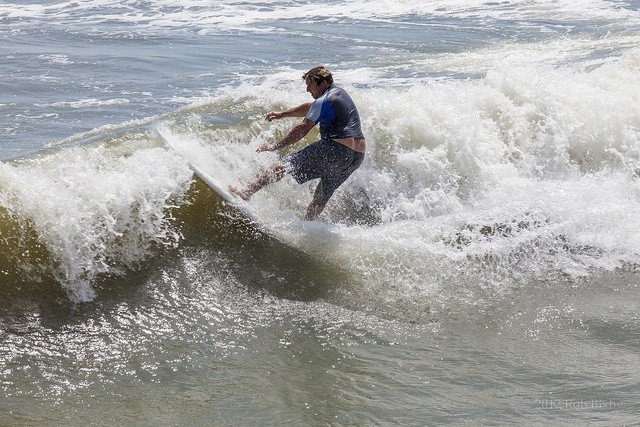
[155,124,307,252]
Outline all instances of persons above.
[228,65,366,222]
